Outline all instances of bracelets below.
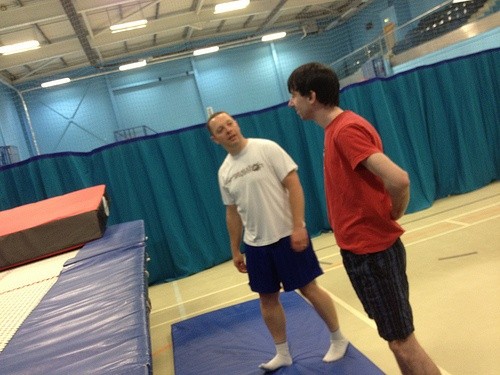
[293,221,306,228]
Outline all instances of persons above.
[286,60,444,375]
[206,110,350,372]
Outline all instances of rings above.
[304,243,307,247]
